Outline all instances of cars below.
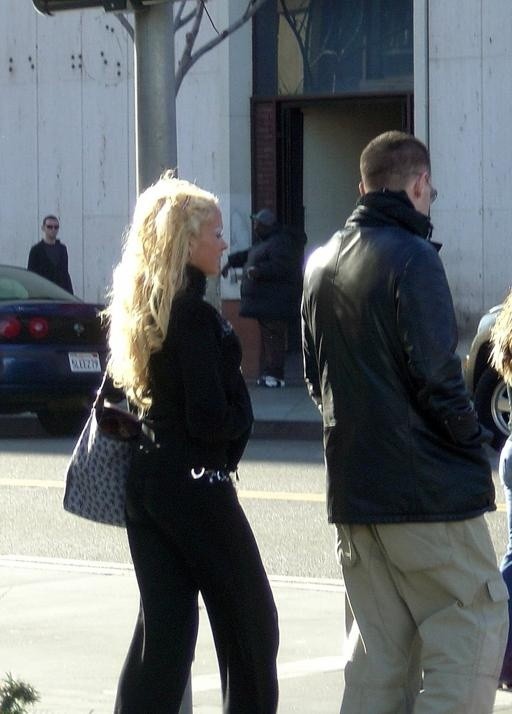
[0,265,109,435]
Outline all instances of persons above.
[489,294,512,691]
[29,215,74,296]
[300,132,509,714]
[108,176,278,714]
[221,210,295,387]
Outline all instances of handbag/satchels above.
[63,404,142,526]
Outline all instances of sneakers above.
[257,375,285,389]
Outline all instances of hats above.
[250,209,275,228]
